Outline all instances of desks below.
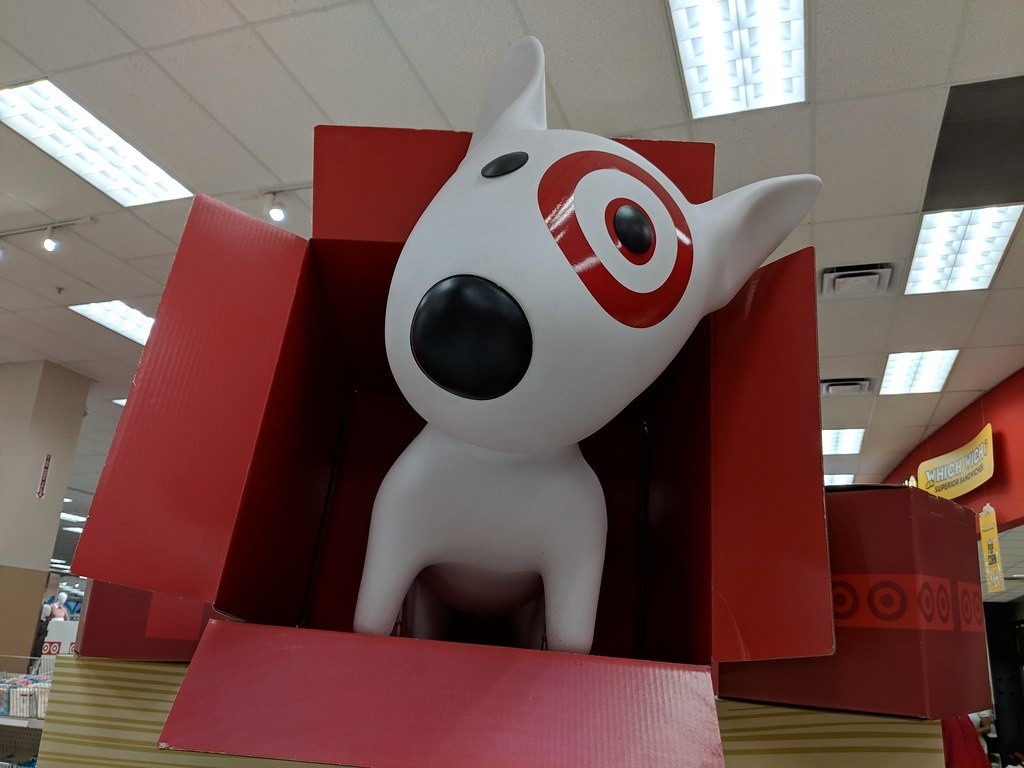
[36,656,950,768]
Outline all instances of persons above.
[27,604,51,675]
[48,591,69,622]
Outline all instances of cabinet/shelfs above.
[0,716,45,768]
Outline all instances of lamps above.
[261,187,287,221]
[36,224,62,251]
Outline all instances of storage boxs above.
[69,118,996,768]
